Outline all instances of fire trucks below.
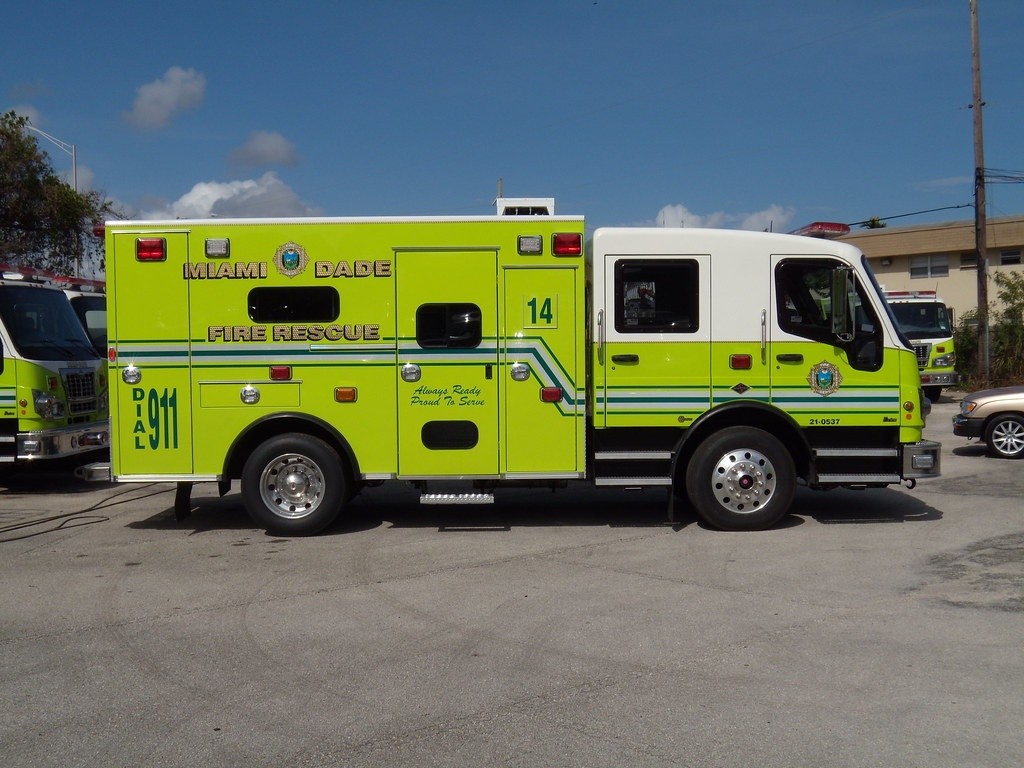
[811,271,960,403]
[0,264,108,471]
[93,214,942,535]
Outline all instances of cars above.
[953,385,1024,458]
